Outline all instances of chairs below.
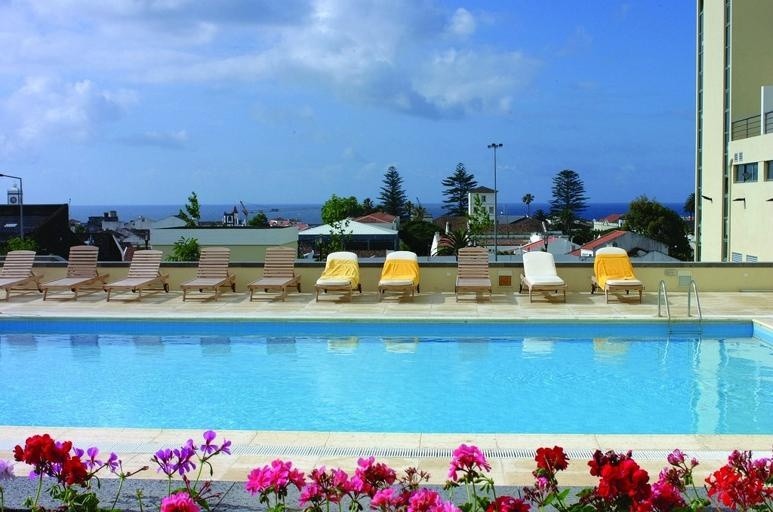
[453,246,493,304]
[326,334,360,359]
[377,336,419,353]
[200,334,231,358]
[5,333,38,352]
[265,336,297,357]
[520,336,555,359]
[178,245,237,303]
[376,249,420,303]
[457,336,491,358]
[313,251,362,304]
[0,250,42,303]
[244,247,302,305]
[593,336,633,361]
[70,334,101,358]
[134,334,164,356]
[102,248,169,303]
[36,245,109,305]
[516,249,567,306]
[589,246,643,306]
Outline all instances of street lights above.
[0,173,24,240]
[144,231,148,249]
[543,232,547,251]
[484,142,505,262]
[318,233,323,262]
